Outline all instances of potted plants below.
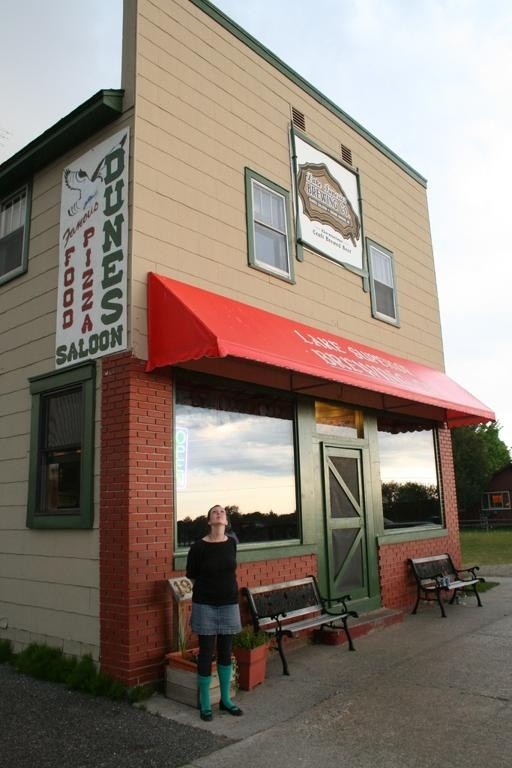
[164,647,241,707]
[230,623,273,691]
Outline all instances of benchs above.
[405,552,486,619]
[239,574,359,676]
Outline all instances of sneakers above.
[198,703,213,721]
[218,697,243,716]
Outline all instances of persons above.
[186,504,244,721]
